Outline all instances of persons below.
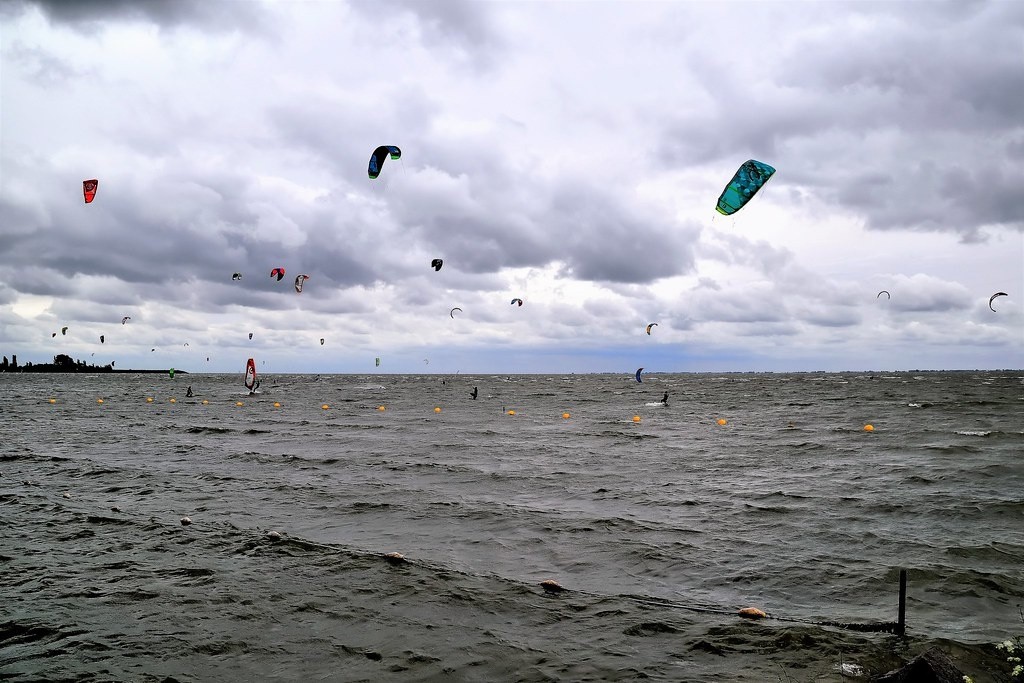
[186,386,193,397]
[661,391,669,404]
[470,387,478,399]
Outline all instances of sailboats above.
[245,358,262,396]
